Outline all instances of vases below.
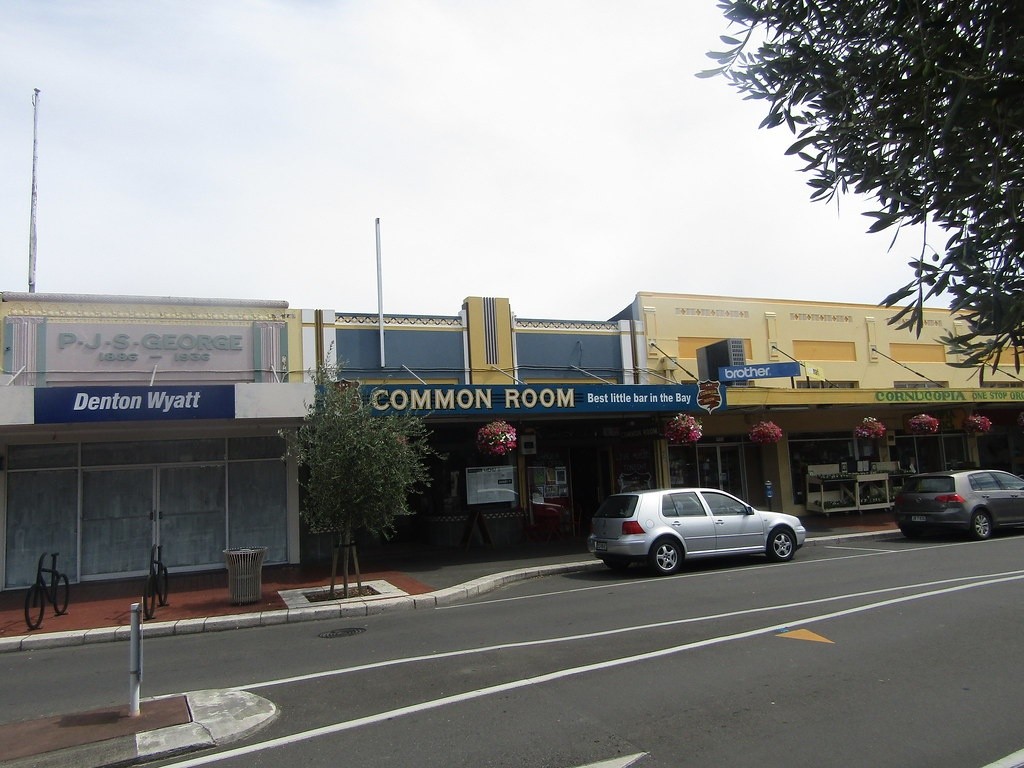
[969,430,984,438]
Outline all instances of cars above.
[587,487,807,576]
[893,468,1024,541]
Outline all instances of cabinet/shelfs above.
[804,464,917,518]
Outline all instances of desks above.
[533,509,572,545]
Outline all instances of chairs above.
[662,495,735,517]
[512,503,568,553]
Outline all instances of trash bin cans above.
[222,547,265,607]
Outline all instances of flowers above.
[909,412,937,434]
[749,420,783,446]
[961,414,990,438]
[663,412,703,447]
[474,420,516,457]
[855,416,886,441]
